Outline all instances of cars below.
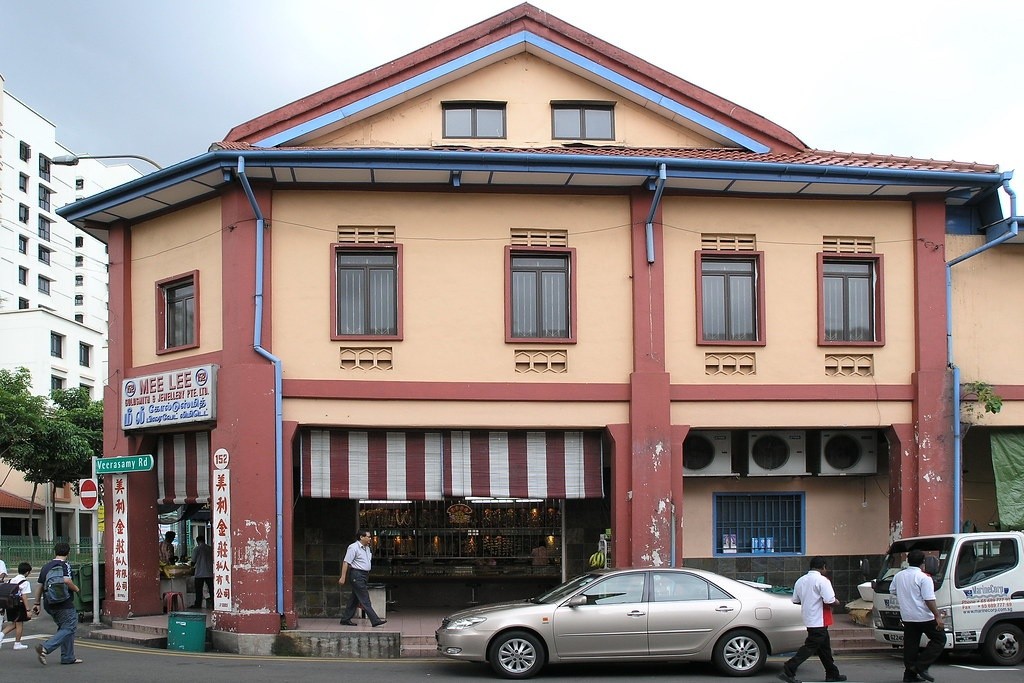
[435,567,808,679]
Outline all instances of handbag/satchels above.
[823,603,833,626]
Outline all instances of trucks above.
[860,530,1024,666]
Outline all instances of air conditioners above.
[815,429,879,478]
[744,428,813,478]
[681,430,740,479]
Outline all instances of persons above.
[159,531,176,565]
[776,558,847,683]
[889,550,947,683]
[476,549,496,566]
[339,527,387,627]
[32,543,82,665]
[0,549,9,632]
[531,540,556,566]
[188,536,214,610]
[374,541,387,557]
[0,562,32,650]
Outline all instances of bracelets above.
[27,609,31,612]
[34,602,40,605]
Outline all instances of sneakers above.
[0,640,3,648]
[13,642,28,650]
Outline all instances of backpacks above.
[0,579,28,610]
[44,561,70,605]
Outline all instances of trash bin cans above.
[69,563,105,623]
[168,613,207,652]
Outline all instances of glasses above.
[365,536,371,538]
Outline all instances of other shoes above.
[187,604,201,609]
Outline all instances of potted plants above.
[959,379,1003,439]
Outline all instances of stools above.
[162,592,184,614]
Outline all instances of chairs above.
[924,555,940,577]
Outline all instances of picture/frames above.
[723,535,738,553]
[751,538,774,553]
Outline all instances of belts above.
[354,569,370,573]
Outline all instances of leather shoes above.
[372,620,387,627]
[61,658,82,664]
[340,620,357,626]
[36,643,47,665]
[919,669,935,682]
[825,675,847,682]
[903,674,925,682]
[777,669,802,683]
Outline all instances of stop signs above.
[79,478,99,511]
